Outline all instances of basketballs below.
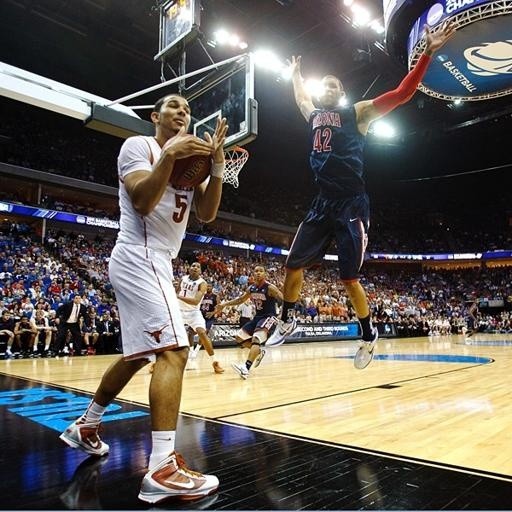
[160,134,211,187]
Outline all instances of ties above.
[76,305,78,319]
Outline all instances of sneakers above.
[59,416,112,456]
[233,363,250,380]
[265,314,298,348]
[354,326,379,369]
[137,450,220,504]
[212,361,224,373]
[254,350,265,368]
